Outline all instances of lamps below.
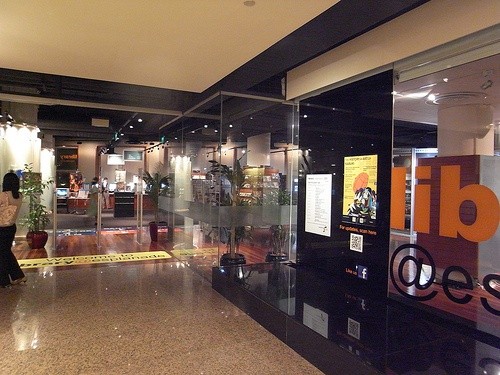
[114,133,120,142]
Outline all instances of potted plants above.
[18,162,56,249]
[142,171,175,241]
[205,149,297,267]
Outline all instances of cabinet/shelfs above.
[114,191,135,218]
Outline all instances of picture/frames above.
[123,149,144,162]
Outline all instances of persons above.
[70,173,83,214]
[0,172,27,288]
[91,177,110,209]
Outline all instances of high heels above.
[0,285,11,290]
[10,277,27,285]
